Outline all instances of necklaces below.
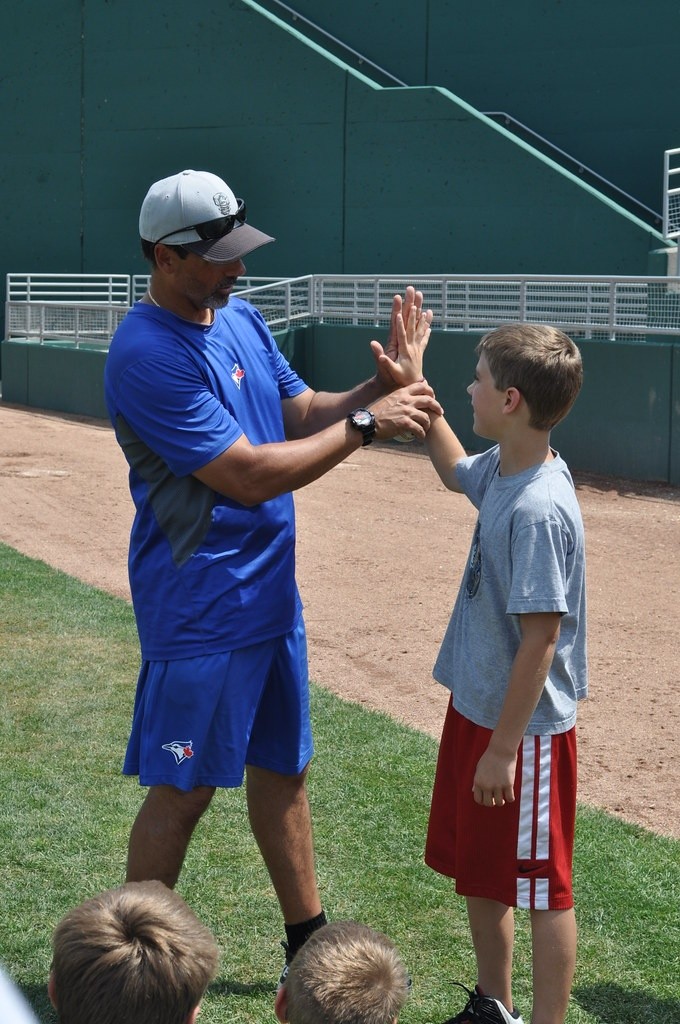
[147,288,162,307]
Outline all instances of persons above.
[48,880,220,1024]
[380,307,590,1024]
[275,923,409,1023]
[103,167,445,993]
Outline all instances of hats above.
[139,169,276,264]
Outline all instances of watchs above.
[348,408,376,448]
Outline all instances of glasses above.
[150,198,248,258]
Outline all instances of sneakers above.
[442,982,524,1024]
